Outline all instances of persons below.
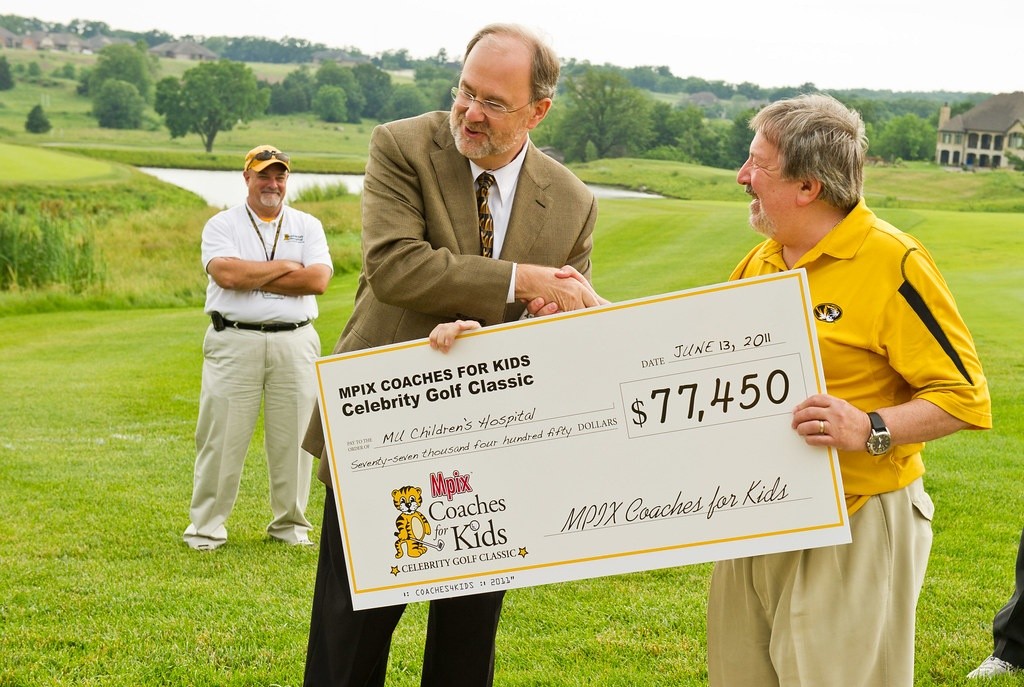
[300,21,596,687]
[181,143,334,550]
[519,93,993,687]
[965,525,1024,682]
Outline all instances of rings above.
[820,420,823,433]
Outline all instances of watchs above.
[866,412,890,456]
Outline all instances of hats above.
[245,145,290,173]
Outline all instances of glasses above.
[449,77,540,119]
[246,150,289,170]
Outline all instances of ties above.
[475,173,494,258]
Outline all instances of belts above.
[222,318,311,332]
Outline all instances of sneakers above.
[965,654,1017,679]
